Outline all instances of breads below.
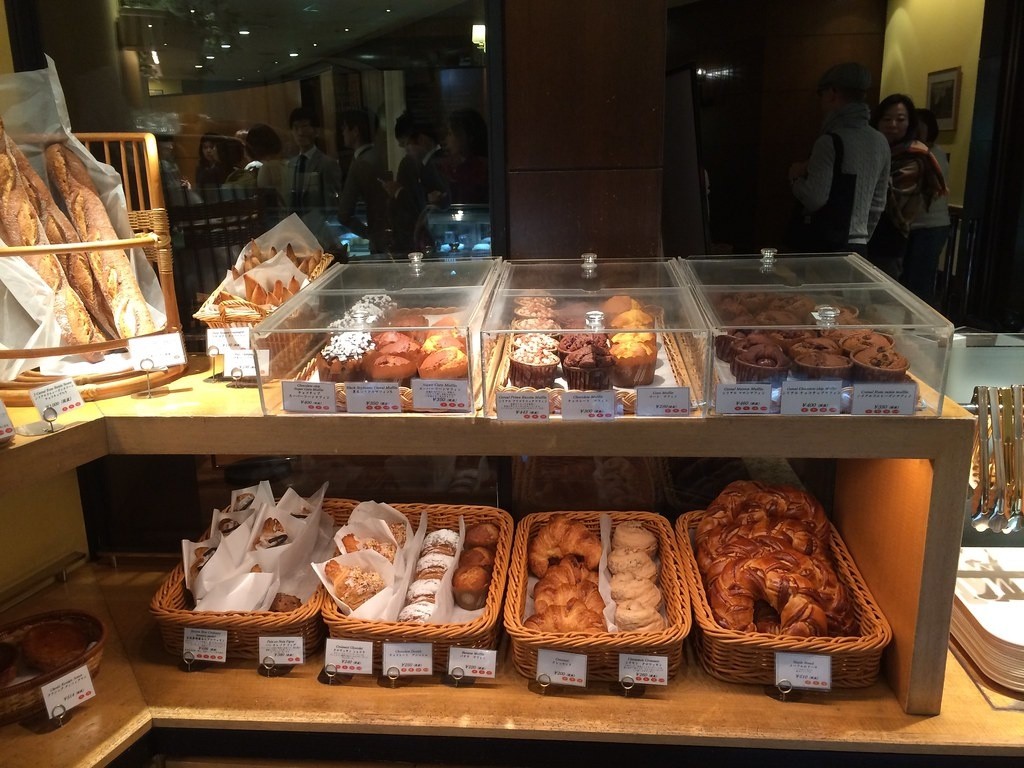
[522,517,666,633]
[323,520,500,624]
[188,493,311,613]
[0,622,91,689]
[695,479,860,639]
[0,114,158,364]
[213,238,323,309]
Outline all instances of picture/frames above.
[926,65,962,132]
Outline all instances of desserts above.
[317,293,468,387]
[711,290,911,391]
[508,294,657,390]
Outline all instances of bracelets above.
[791,177,798,186]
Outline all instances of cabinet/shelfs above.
[91,353,1024,767]
[0,399,165,768]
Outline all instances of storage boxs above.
[678,248,954,419]
[250,253,502,415]
[481,253,711,419]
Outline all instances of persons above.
[154,106,488,336]
[788,62,951,301]
